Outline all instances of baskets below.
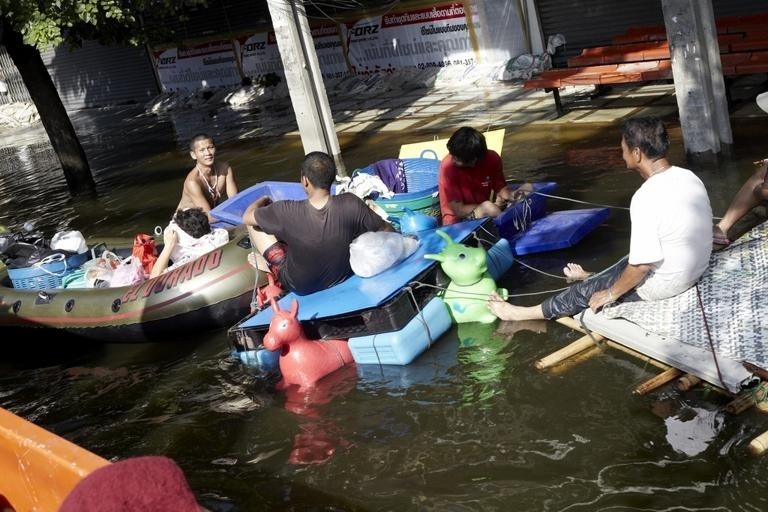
[7,248,92,290]
[353,149,445,232]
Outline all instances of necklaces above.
[195,162,219,199]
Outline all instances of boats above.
[536,215,767,455]
[0,227,275,344]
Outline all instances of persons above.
[439,126,536,226]
[484,116,714,322]
[713,92,768,245]
[243,151,393,297]
[149,208,230,279]
[175,134,238,223]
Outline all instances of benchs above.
[522,13,768,122]
